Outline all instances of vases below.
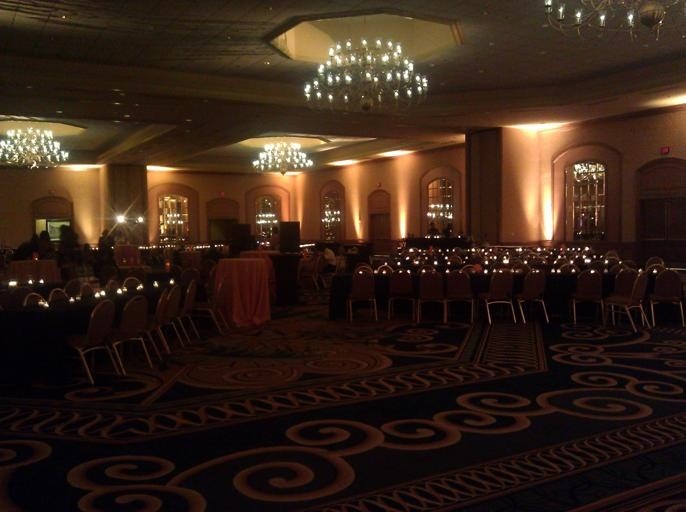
[428,221,439,238]
[443,219,454,238]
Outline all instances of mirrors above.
[552,147,621,240]
[148,181,199,243]
[420,165,461,238]
[244,186,290,248]
[319,179,345,241]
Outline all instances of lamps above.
[255,197,278,248]
[320,200,340,241]
[540,0,686,42]
[426,176,454,225]
[0,115,88,170]
[158,195,184,237]
[262,14,465,114]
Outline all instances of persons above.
[10,225,120,275]
[319,243,337,272]
[271,226,280,250]
[427,222,440,235]
[151,244,222,280]
[442,222,454,235]
